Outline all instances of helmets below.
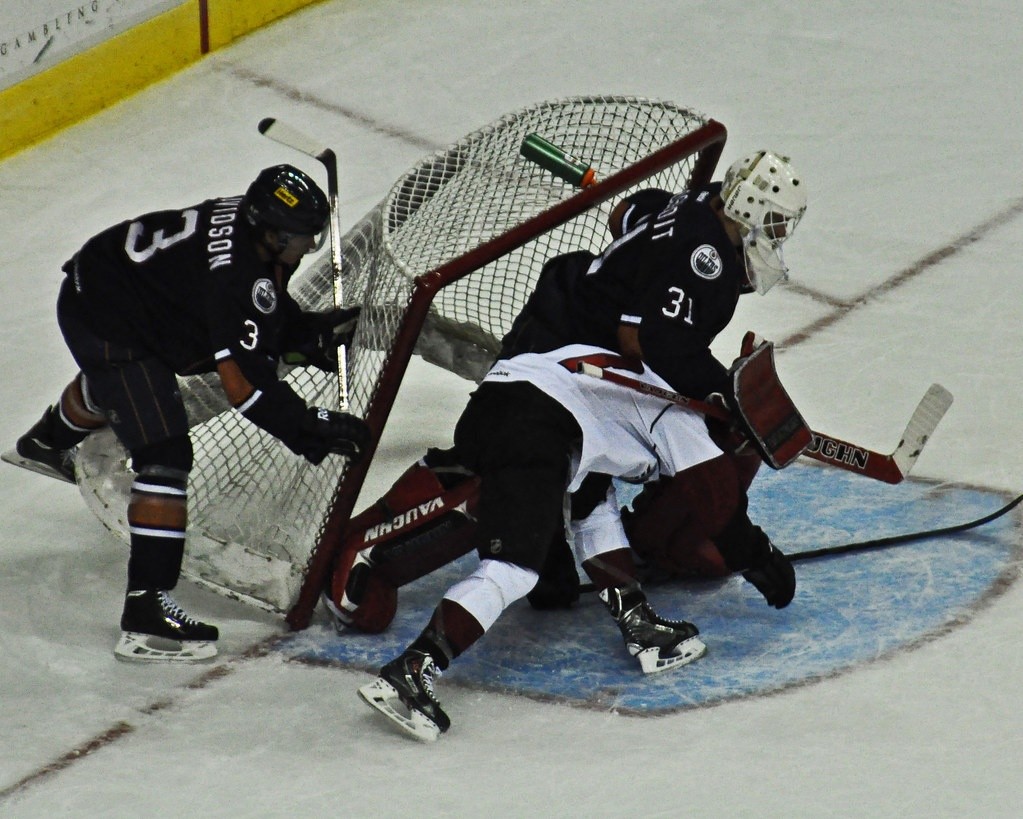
[720,150,806,247]
[243,163,332,254]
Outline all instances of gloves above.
[738,524,796,609]
[285,405,372,466]
[284,307,361,373]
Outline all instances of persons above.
[321,150,810,745]
[0,164,370,665]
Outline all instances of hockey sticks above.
[259,117,350,414]
[578,361,954,487]
[789,492,1023,562]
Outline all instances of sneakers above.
[357,647,451,744]
[114,587,219,661]
[1,405,92,485]
[600,582,707,674]
